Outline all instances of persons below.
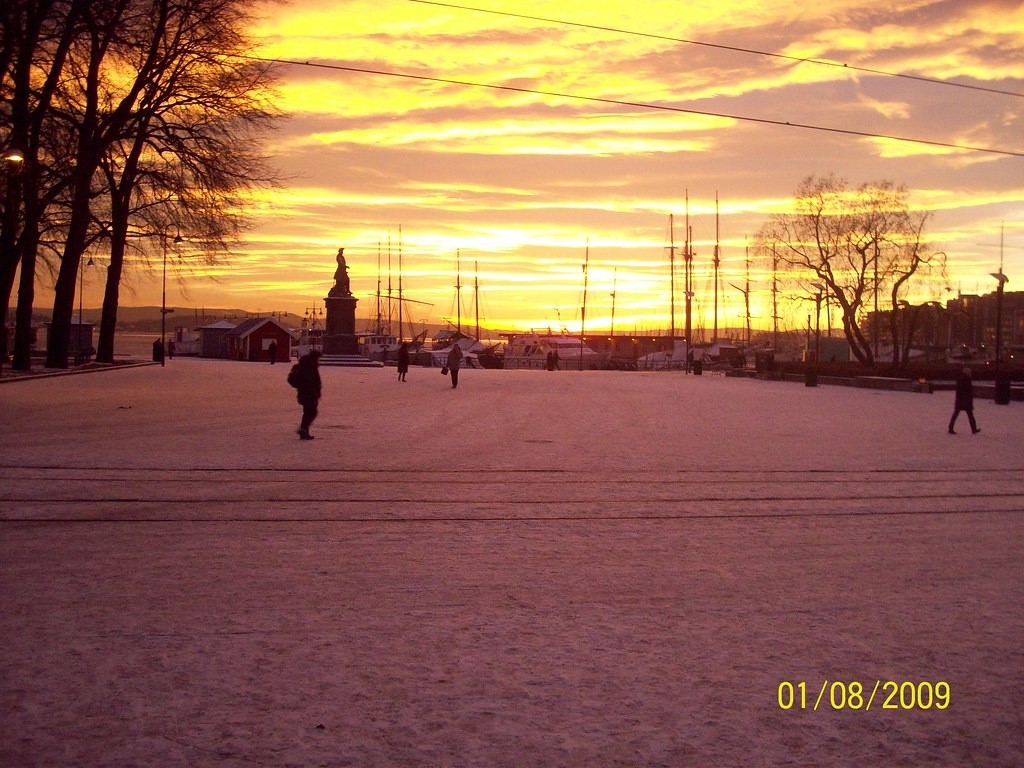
[445,344,463,389]
[295,350,323,440]
[688,350,694,373]
[168,339,176,360]
[397,342,409,382]
[544,350,561,371]
[268,340,277,364]
[948,366,981,435]
[334,248,353,294]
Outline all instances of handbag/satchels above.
[441,367,448,375]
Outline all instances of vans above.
[291,337,323,356]
[889,360,941,378]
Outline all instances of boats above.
[358,336,417,363]
[500,338,600,369]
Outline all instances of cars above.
[781,362,831,381]
[927,345,938,352]
[835,361,873,378]
[874,351,909,362]
[982,360,1024,381]
[938,349,992,378]
[74,346,96,356]
[875,366,903,378]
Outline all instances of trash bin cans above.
[153,344,163,362]
[693,360,702,375]
[995,373,1012,404]
[804,367,817,387]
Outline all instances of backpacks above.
[288,359,306,388]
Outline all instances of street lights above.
[305,302,323,350]
[161,223,186,366]
[990,273,1009,368]
[78,250,94,347]
[224,313,237,324]
[271,310,288,323]
[204,313,217,324]
[810,282,827,362]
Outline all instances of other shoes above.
[402,380,406,382]
[948,430,958,434]
[296,429,314,441]
[972,428,981,434]
